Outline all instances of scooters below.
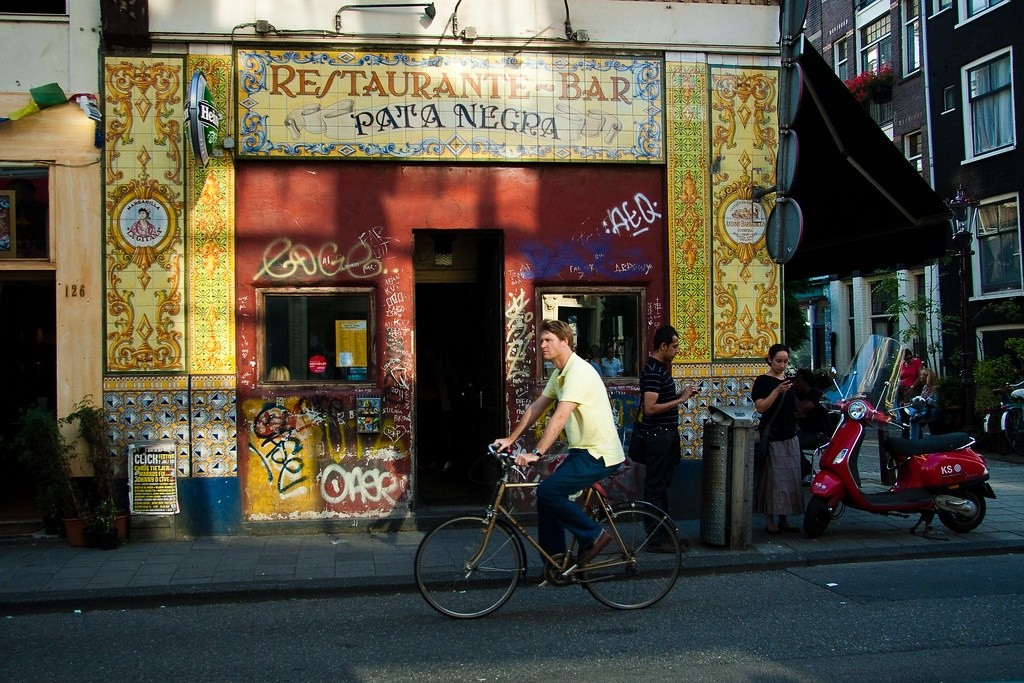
[798,334,995,542]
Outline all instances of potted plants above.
[7,393,130,548]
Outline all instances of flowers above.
[843,63,894,104]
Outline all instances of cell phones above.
[785,376,796,386]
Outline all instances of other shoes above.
[765,525,783,536]
[778,522,800,533]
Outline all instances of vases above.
[879,81,893,101]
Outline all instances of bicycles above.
[412,442,689,618]
[982,381,1024,458]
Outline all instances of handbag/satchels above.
[754,433,770,466]
[628,420,681,468]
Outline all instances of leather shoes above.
[531,574,570,588]
[578,528,613,565]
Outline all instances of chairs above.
[802,409,845,486]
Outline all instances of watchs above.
[533,448,542,457]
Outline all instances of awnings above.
[784,35,953,283]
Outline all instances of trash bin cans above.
[701,406,758,548]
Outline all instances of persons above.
[750,344,804,535]
[581,345,624,377]
[493,318,626,584]
[794,368,832,450]
[639,325,700,554]
[897,348,923,440]
[904,369,937,439]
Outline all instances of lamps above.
[572,29,590,41]
